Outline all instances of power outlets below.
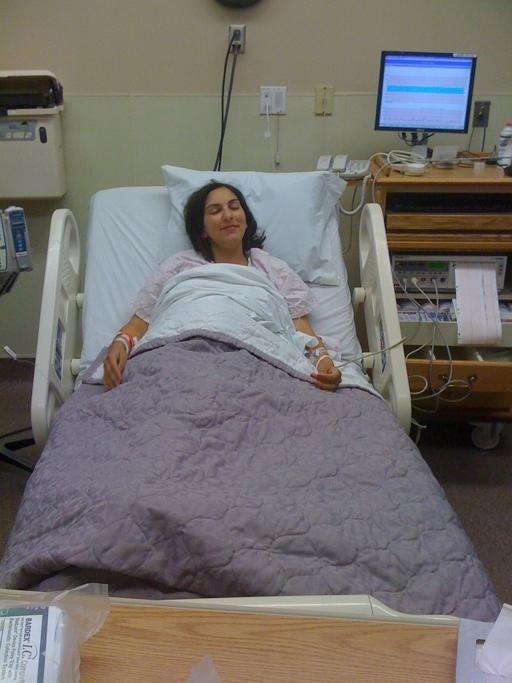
[227,24,246,53]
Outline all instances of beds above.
[0,185,512,683]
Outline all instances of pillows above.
[157,163,348,288]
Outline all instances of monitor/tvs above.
[374,50,478,134]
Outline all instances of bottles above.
[496,122,512,168]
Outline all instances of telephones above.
[330,154,370,181]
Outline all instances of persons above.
[100,176,343,397]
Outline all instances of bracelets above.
[111,330,136,358]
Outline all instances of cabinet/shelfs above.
[346,149,512,423]
